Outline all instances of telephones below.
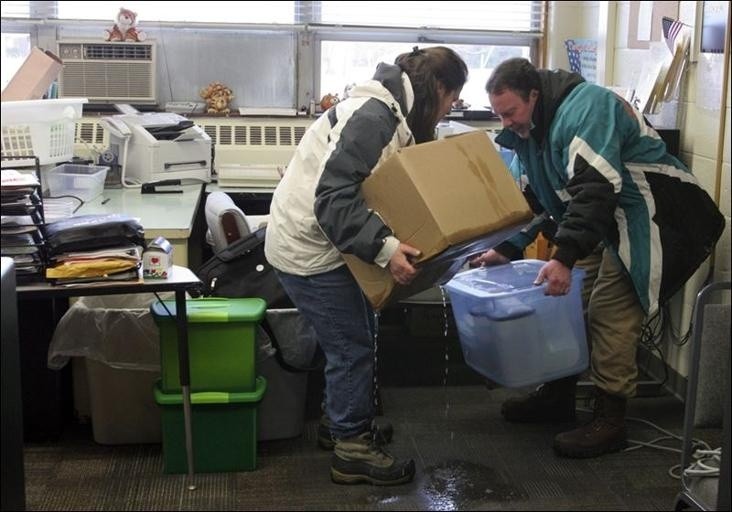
[97,116,132,139]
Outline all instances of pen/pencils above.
[102,198,110,204]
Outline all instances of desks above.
[43,175,277,269]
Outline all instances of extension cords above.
[165,101,206,114]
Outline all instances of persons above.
[263,46,469,486]
[471,58,725,459]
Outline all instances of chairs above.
[670,281,731,511]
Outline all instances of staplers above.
[141,179,183,194]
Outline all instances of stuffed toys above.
[103,7,147,43]
[321,93,340,110]
[199,81,233,114]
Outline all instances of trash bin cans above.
[247,304,312,442]
[153,383,266,472]
[149,298,269,392]
[78,292,188,447]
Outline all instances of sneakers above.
[330,419,415,486]
[318,415,395,450]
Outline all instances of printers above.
[100,112,211,186]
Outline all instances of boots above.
[554,384,628,458]
[500,376,577,424]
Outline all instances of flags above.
[663,17,683,57]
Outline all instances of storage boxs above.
[150,298,268,472]
[342,130,533,315]
[441,257,590,387]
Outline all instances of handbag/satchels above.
[196,225,288,310]
